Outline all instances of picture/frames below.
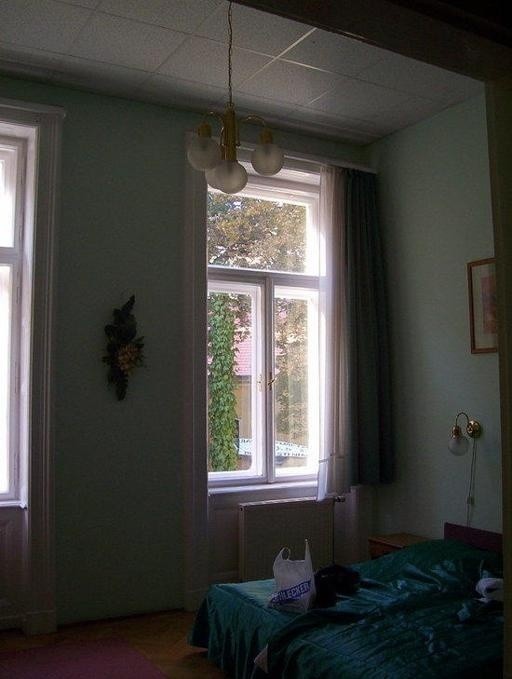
[449,411,482,456]
[468,257,501,354]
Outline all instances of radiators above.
[239,495,335,584]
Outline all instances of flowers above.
[100,293,149,390]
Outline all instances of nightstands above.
[368,531,427,562]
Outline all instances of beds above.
[205,522,504,678]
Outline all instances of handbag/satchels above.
[320,559,360,596]
[313,567,337,609]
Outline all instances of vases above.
[108,366,128,400]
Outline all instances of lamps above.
[188,0,283,194]
